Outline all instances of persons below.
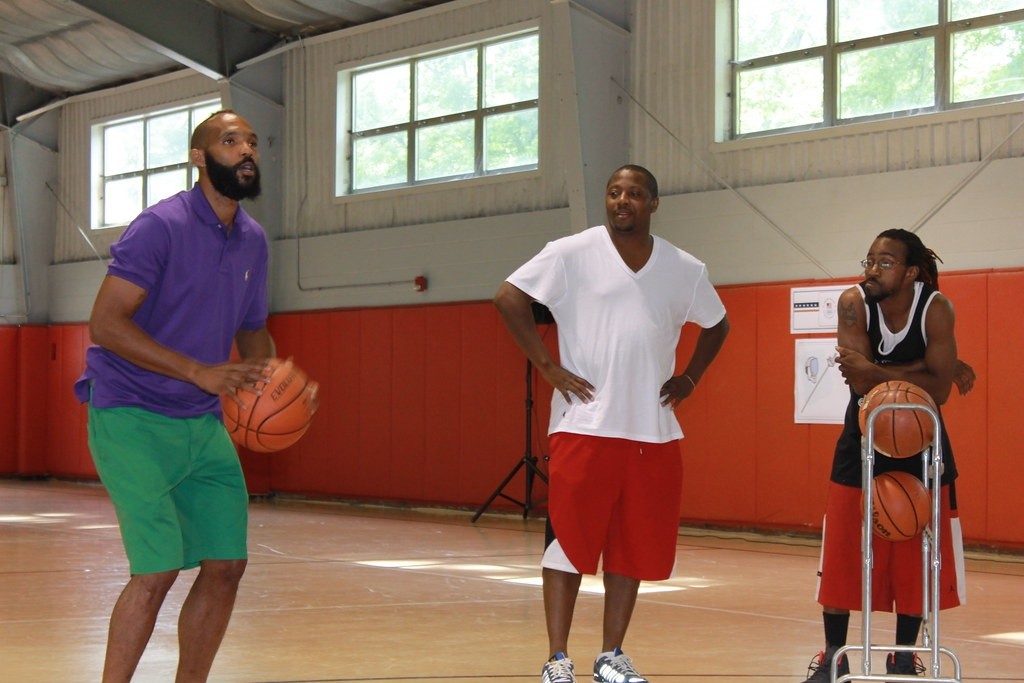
[493,163,730,683]
[70,108,314,683]
[804,228,977,683]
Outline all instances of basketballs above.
[221,359,317,454]
[857,380,939,458]
[860,469,932,541]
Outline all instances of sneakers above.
[541,652,576,683]
[592,647,648,683]
[801,650,853,683]
[886,652,917,675]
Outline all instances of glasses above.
[860,258,905,271]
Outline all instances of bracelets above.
[683,373,695,391]
